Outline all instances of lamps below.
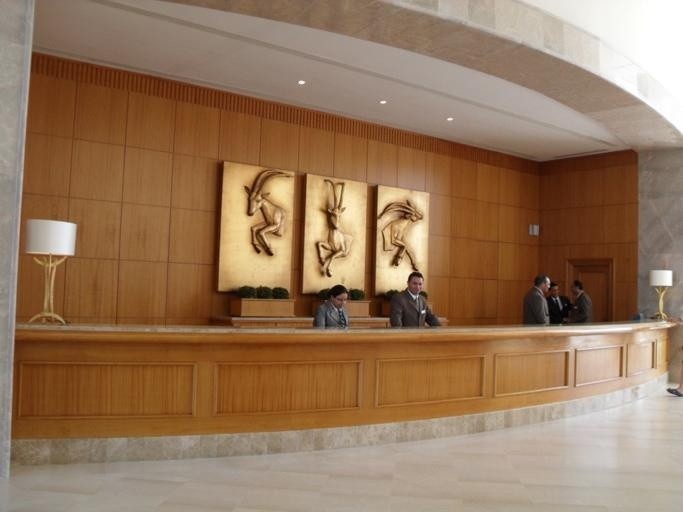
[648,270,673,319]
[24,218,77,325]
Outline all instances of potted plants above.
[229,285,297,318]
[380,289,433,317]
[312,289,372,318]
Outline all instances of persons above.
[390,272,441,328]
[316,285,348,328]
[523,276,551,324]
[568,280,592,322]
[667,367,683,396]
[546,283,574,324]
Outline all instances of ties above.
[555,299,560,310]
[338,309,346,327]
